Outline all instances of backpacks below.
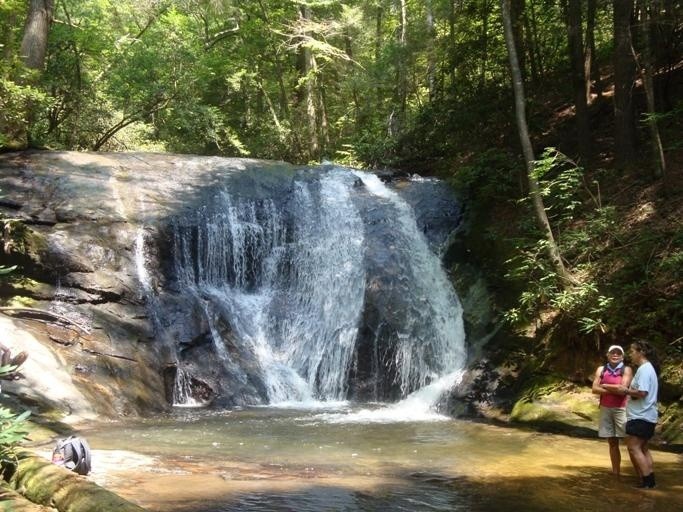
[52,435,91,475]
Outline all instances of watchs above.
[623,389,629,394]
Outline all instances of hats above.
[609,345,624,355]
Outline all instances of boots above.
[637,473,656,488]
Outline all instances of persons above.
[590,343,643,486]
[611,339,660,489]
